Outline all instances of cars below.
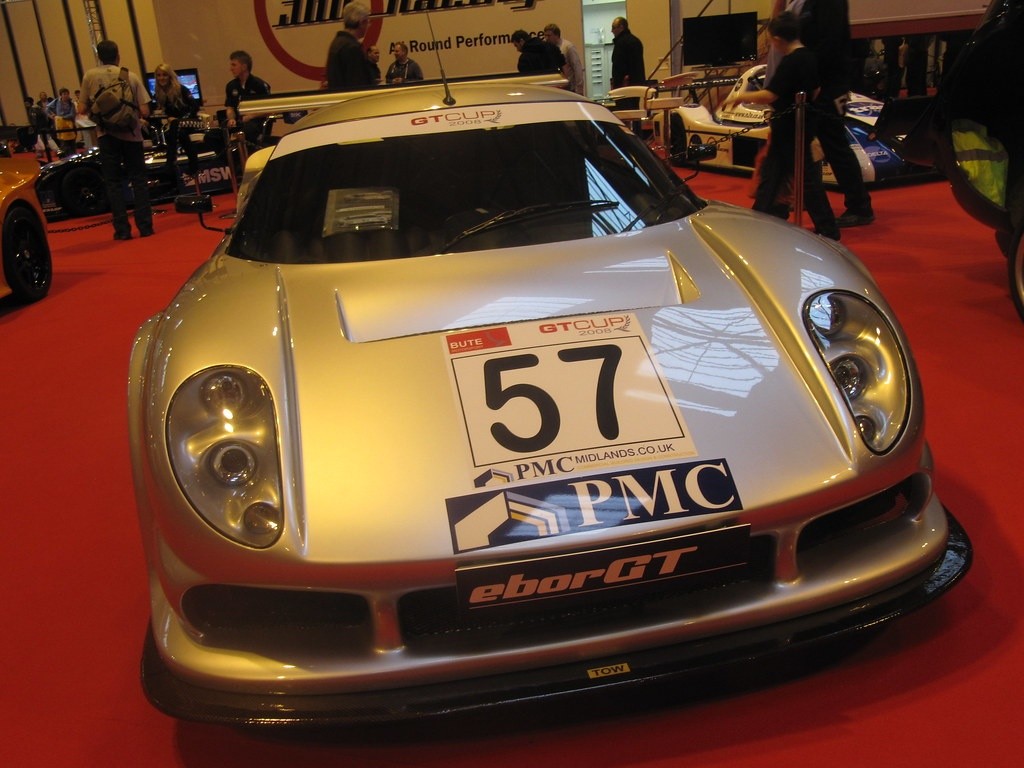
[0,155,55,301]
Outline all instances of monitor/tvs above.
[682,11,758,68]
[143,68,204,108]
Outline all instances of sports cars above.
[652,63,949,189]
[125,71,973,732]
[34,132,267,217]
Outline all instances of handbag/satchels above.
[88,66,139,134]
[54,115,78,142]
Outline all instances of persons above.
[387,42,424,83]
[545,23,584,95]
[327,2,375,90]
[611,18,645,139]
[764,0,875,227]
[511,30,575,91]
[24,88,96,158]
[154,63,199,123]
[750,13,841,240]
[221,51,272,177]
[9,122,34,153]
[77,44,154,241]
[367,46,382,82]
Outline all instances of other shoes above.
[835,210,873,227]
[814,231,840,241]
[139,229,154,237]
[113,231,131,241]
